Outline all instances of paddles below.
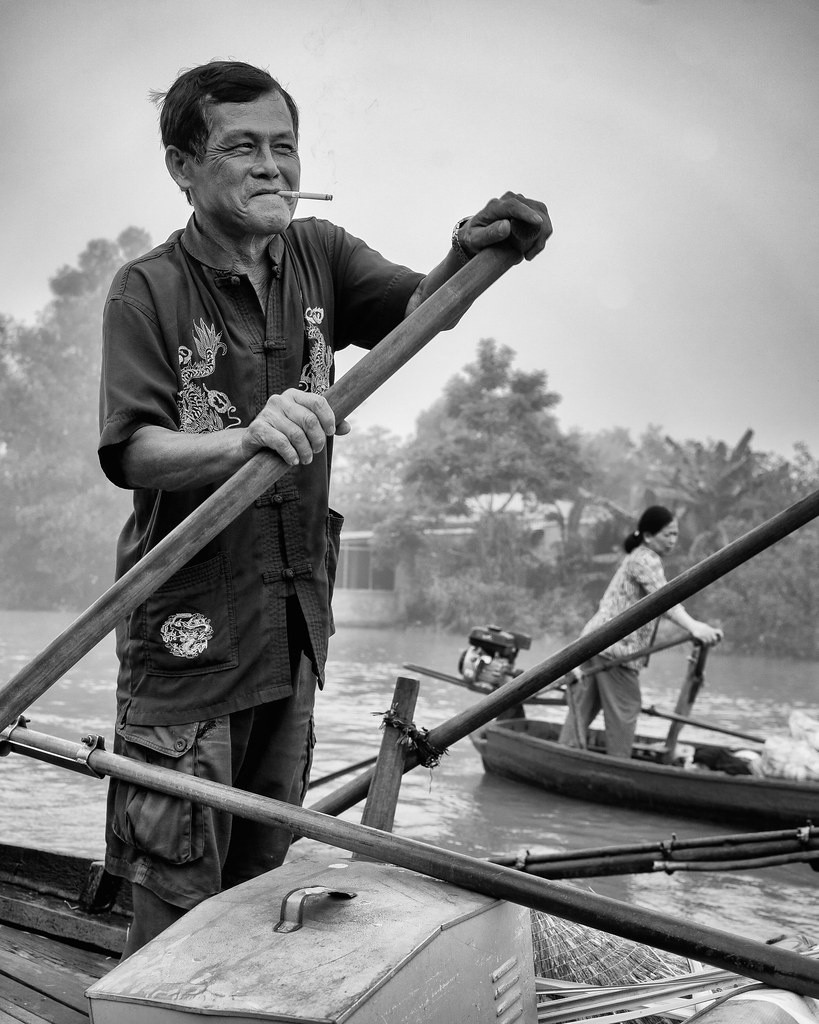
[0,218,819,1001]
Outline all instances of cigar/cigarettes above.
[277,191,333,203]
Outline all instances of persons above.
[98,63,554,964]
[555,505,722,759]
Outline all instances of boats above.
[458,626,819,833]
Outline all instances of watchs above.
[451,216,470,263]
